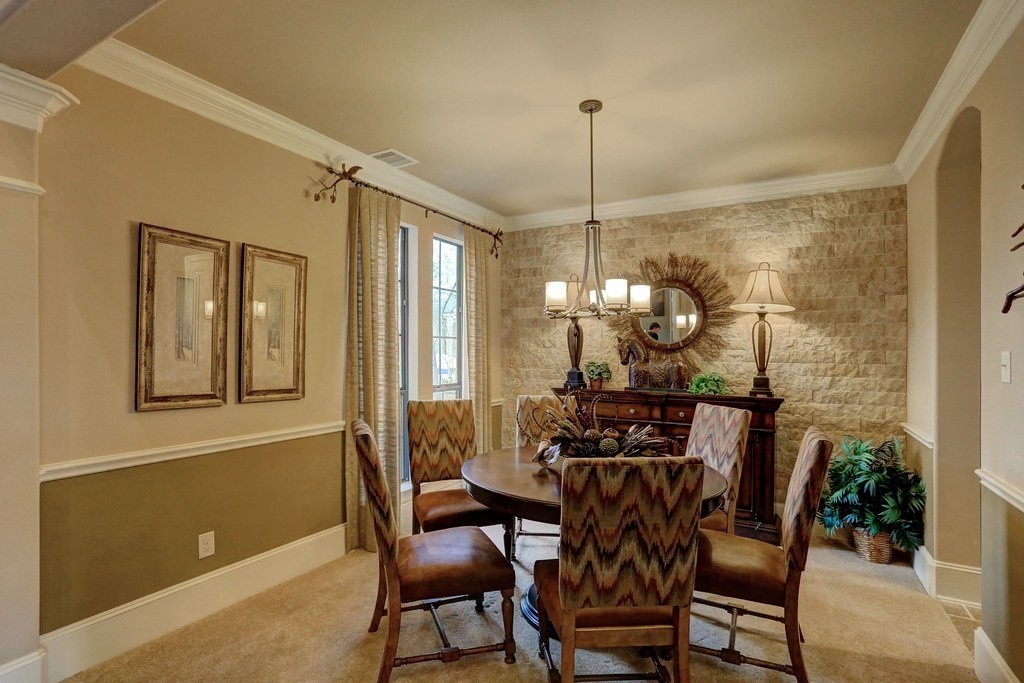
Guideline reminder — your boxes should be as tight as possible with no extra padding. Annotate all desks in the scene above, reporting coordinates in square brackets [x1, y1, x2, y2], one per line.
[461, 445, 730, 563]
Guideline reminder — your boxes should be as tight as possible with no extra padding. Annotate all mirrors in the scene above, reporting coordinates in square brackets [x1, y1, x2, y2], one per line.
[629, 277, 705, 351]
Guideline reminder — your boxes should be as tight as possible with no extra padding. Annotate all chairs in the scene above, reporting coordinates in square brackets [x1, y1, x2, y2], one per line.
[686, 402, 753, 535]
[694, 425, 834, 683]
[534, 456, 706, 683]
[350, 417, 520, 683]
[511, 395, 577, 561]
[409, 398, 512, 564]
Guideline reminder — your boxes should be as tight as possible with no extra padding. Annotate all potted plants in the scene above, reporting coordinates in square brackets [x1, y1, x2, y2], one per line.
[688, 372, 731, 396]
[584, 360, 612, 390]
[817, 434, 926, 565]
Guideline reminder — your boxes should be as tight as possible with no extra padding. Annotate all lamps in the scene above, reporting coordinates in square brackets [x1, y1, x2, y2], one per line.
[676, 314, 696, 329]
[543, 99, 655, 321]
[550, 273, 594, 389]
[732, 261, 797, 396]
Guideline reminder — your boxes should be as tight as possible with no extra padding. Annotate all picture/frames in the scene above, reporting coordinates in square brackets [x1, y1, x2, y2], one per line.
[133, 221, 232, 415]
[238, 241, 310, 403]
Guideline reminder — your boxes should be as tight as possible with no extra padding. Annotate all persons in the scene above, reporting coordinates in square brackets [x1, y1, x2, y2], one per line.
[648, 322, 661, 340]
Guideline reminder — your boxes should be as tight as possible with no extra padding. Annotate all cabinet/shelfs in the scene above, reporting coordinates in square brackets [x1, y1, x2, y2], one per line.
[550, 386, 786, 544]
